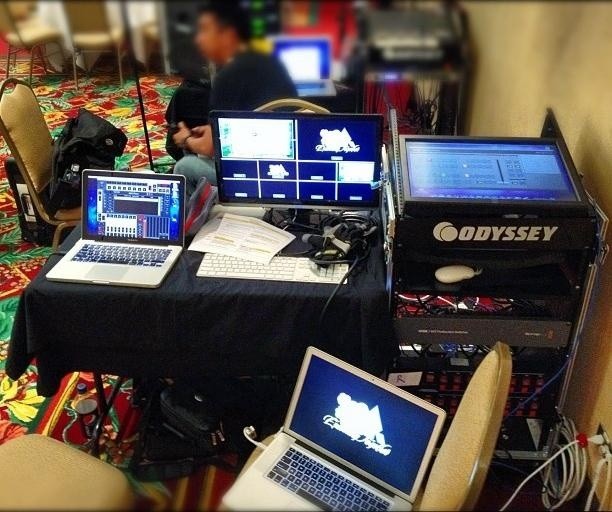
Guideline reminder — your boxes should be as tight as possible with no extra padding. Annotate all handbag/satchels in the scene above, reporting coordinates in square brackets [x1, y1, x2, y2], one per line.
[159, 380, 225, 449]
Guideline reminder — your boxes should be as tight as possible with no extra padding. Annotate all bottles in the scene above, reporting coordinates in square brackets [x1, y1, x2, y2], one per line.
[71, 384, 99, 441]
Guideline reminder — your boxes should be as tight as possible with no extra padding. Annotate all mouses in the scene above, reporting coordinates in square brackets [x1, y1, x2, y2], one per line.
[434, 264, 475, 284]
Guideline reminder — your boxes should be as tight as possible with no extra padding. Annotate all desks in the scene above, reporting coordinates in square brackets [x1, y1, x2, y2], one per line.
[5, 210, 382, 459]
[37, 0, 158, 75]
[165, 81, 356, 164]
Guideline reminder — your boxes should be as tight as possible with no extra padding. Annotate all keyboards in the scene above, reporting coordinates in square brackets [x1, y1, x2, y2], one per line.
[195, 249, 348, 284]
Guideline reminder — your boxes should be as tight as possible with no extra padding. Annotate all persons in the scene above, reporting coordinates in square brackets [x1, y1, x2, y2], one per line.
[175, 8, 297, 210]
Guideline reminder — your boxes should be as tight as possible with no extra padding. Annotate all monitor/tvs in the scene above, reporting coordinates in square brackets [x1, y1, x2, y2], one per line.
[206, 107, 385, 226]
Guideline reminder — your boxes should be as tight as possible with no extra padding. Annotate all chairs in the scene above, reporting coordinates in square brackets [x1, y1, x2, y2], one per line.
[219, 341, 512, 512]
[62, 0, 129, 89]
[0, 0, 67, 84]
[0, 433, 134, 512]
[0, 77, 82, 252]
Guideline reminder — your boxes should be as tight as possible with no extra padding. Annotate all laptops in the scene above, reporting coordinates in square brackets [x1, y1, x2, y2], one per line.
[46, 168, 185, 288]
[269, 36, 334, 95]
[220, 346, 446, 512]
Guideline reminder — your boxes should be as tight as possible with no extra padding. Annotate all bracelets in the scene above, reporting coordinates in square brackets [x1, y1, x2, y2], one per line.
[182, 135, 192, 150]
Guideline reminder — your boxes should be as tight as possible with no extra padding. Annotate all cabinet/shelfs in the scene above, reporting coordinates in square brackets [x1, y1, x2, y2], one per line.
[360, 57, 467, 138]
[380, 143, 610, 473]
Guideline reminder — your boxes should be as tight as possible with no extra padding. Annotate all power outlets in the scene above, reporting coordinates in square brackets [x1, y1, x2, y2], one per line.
[593, 422, 612, 466]
